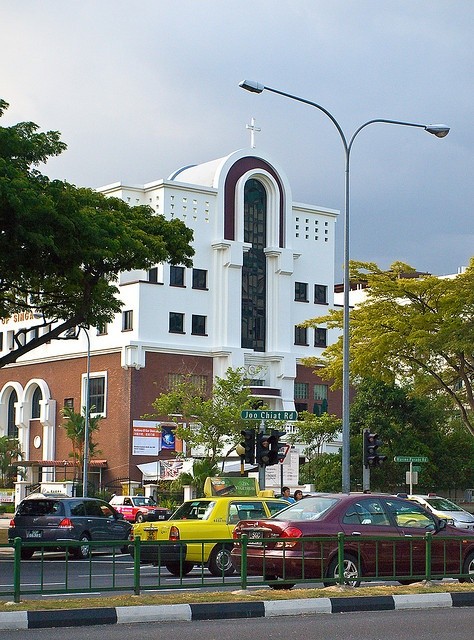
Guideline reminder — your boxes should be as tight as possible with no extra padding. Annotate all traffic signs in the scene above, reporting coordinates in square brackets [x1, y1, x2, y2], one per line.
[393, 455, 429, 463]
[239, 409, 299, 421]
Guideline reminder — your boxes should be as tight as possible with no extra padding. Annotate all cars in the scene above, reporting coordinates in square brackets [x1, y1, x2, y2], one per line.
[371, 492, 474, 531]
[229, 491, 474, 590]
[128, 497, 290, 575]
[99, 495, 171, 522]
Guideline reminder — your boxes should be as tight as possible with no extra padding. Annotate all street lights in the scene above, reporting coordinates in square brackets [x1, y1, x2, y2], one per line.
[233, 80, 449, 490]
[33, 312, 91, 498]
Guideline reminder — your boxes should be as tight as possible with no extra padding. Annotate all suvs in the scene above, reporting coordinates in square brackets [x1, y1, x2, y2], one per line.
[6, 497, 132, 559]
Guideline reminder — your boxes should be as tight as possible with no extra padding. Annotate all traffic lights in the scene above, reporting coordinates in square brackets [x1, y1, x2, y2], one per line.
[364, 429, 378, 469]
[256, 432, 274, 466]
[271, 430, 286, 464]
[239, 428, 254, 464]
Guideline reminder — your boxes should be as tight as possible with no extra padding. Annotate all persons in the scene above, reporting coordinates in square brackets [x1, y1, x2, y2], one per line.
[279, 487, 295, 505]
[294, 490, 303, 502]
